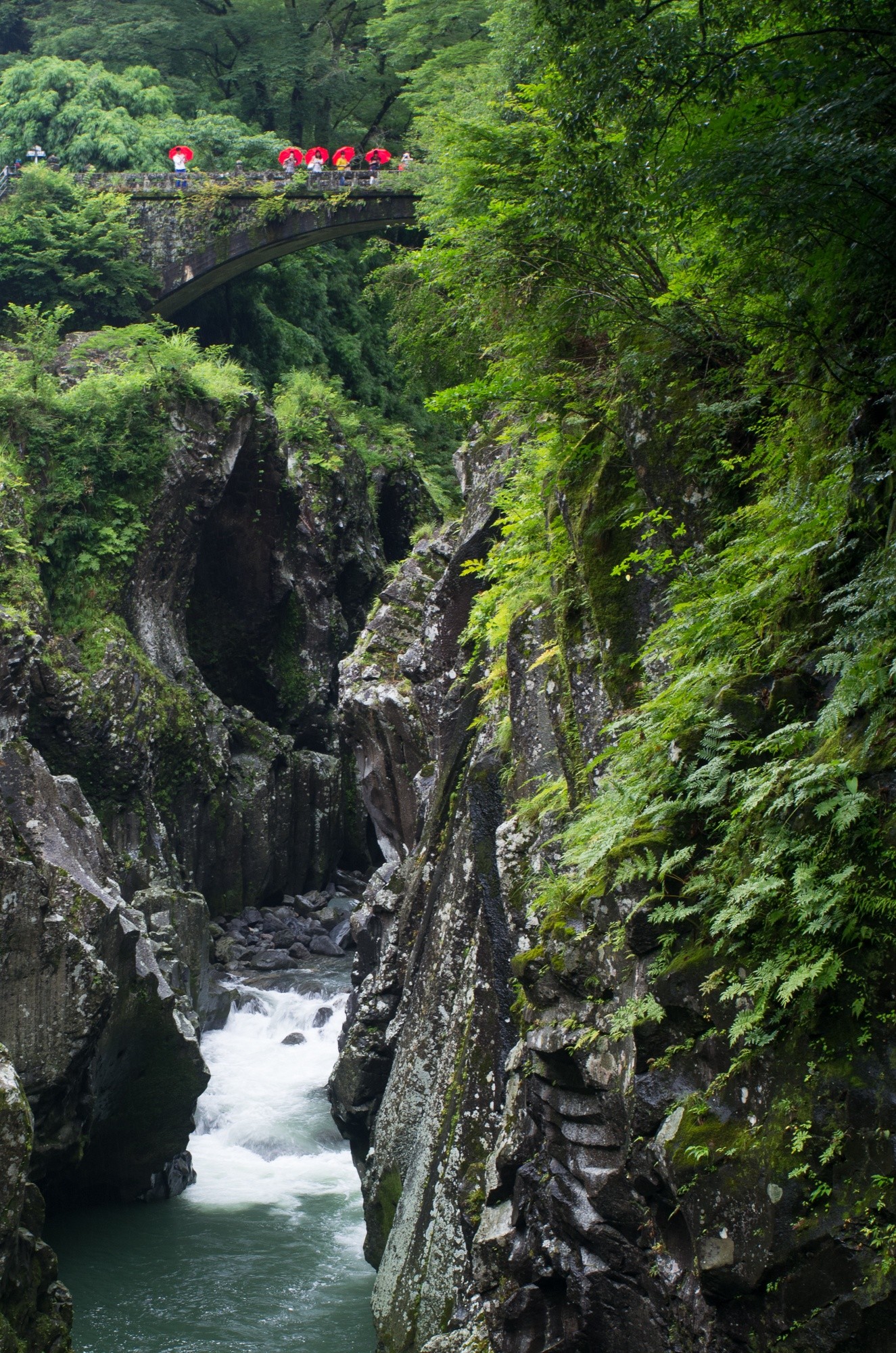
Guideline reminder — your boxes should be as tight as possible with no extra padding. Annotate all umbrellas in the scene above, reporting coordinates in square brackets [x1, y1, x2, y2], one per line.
[170, 146, 194, 163]
[304, 147, 329, 164]
[332, 146, 355, 165]
[278, 147, 303, 166]
[365, 148, 391, 164]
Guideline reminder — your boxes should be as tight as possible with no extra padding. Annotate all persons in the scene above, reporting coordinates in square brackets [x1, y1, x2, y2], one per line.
[14, 158, 22, 168]
[370, 150, 381, 171]
[336, 150, 351, 170]
[283, 151, 298, 182]
[307, 150, 323, 173]
[401, 152, 414, 169]
[173, 148, 188, 187]
